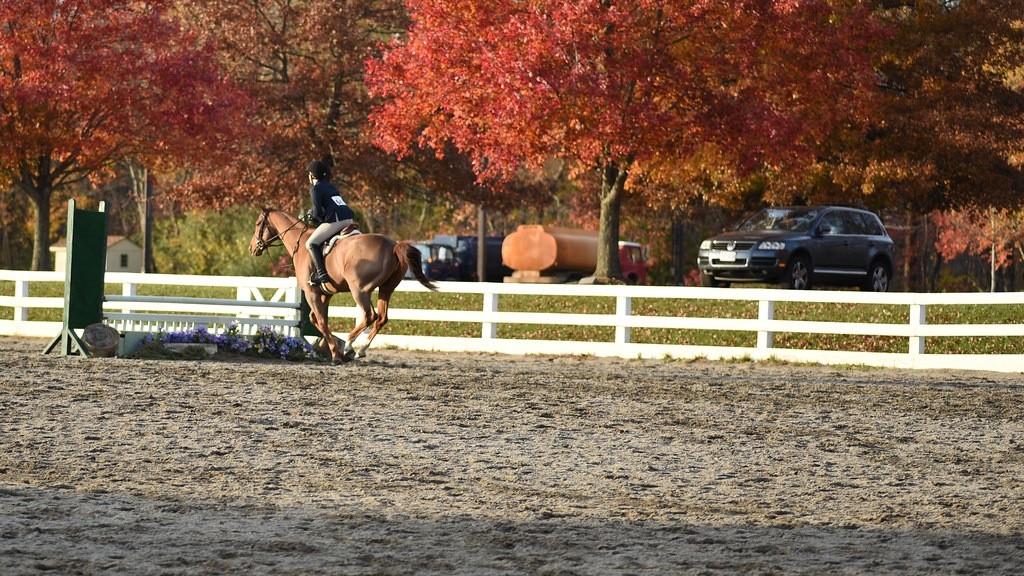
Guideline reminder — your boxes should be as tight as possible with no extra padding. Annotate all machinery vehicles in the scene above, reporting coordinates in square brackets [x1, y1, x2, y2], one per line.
[501, 225, 648, 285]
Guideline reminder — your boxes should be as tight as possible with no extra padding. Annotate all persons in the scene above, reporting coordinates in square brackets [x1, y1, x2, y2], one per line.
[306, 161, 357, 285]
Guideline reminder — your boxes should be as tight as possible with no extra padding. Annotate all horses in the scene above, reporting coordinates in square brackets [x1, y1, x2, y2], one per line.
[249, 201, 440, 367]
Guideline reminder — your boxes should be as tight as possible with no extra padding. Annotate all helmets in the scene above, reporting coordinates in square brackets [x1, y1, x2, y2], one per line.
[306, 160, 330, 180]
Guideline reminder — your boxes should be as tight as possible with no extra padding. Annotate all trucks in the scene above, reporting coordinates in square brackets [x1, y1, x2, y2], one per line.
[404, 235, 514, 282]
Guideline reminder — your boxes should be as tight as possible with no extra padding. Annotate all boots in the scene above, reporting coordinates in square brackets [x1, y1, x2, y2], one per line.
[308, 243, 330, 283]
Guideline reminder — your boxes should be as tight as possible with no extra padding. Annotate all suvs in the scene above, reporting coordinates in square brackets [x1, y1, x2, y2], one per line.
[697, 205, 897, 292]
